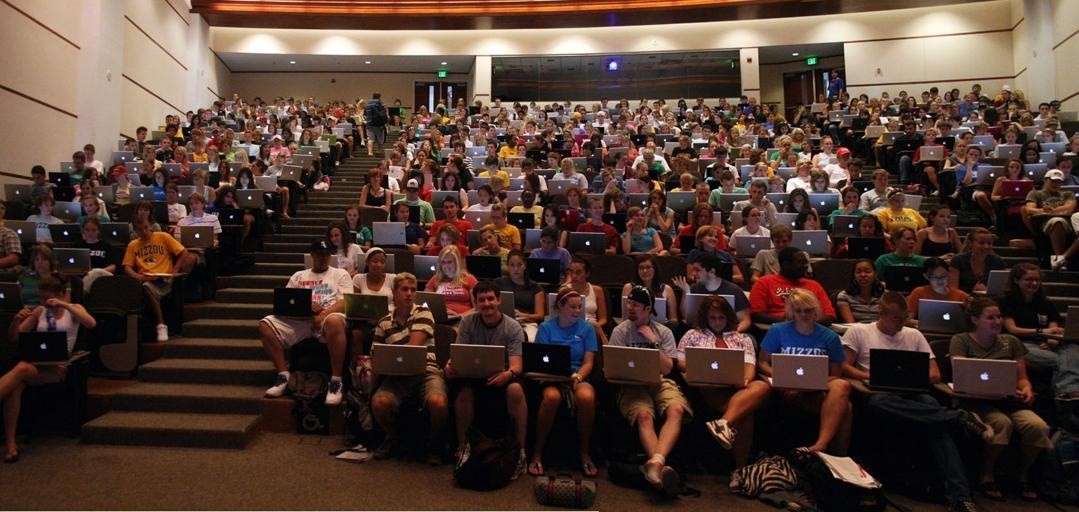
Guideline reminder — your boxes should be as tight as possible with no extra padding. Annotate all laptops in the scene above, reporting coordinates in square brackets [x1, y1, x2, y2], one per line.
[776, 213, 798, 230]
[52, 186, 75, 200]
[584, 113, 596, 122]
[501, 168, 521, 178]
[469, 190, 495, 206]
[1033, 120, 1046, 130]
[389, 205, 421, 222]
[667, 192, 696, 212]
[343, 294, 389, 321]
[360, 208, 386, 229]
[236, 189, 264, 208]
[730, 149, 739, 162]
[548, 293, 588, 322]
[848, 236, 885, 258]
[693, 143, 708, 155]
[332, 128, 343, 139]
[470, 291, 528, 323]
[593, 148, 602, 160]
[99, 222, 131, 244]
[852, 118, 870, 130]
[189, 163, 209, 174]
[630, 194, 651, 208]
[180, 226, 213, 250]
[973, 271, 1012, 296]
[466, 212, 492, 229]
[52, 248, 92, 275]
[1040, 142, 1067, 160]
[551, 117, 561, 124]
[507, 157, 525, 165]
[569, 233, 606, 253]
[94, 186, 113, 203]
[809, 194, 838, 216]
[830, 215, 861, 237]
[573, 157, 586, 172]
[48, 223, 87, 244]
[496, 128, 506, 136]
[254, 176, 277, 192]
[1029, 212, 1072, 223]
[995, 144, 1022, 160]
[971, 135, 993, 152]
[467, 231, 500, 254]
[747, 135, 759, 145]
[648, 169, 658, 180]
[548, 180, 571, 197]
[720, 194, 750, 213]
[149, 202, 169, 224]
[916, 130, 926, 137]
[470, 128, 480, 136]
[415, 149, 422, 156]
[314, 140, 329, 153]
[768, 194, 791, 212]
[553, 149, 571, 158]
[130, 188, 153, 204]
[3, 184, 32, 203]
[769, 354, 828, 390]
[113, 151, 133, 164]
[767, 148, 780, 160]
[510, 120, 523, 127]
[913, 299, 967, 335]
[373, 345, 428, 376]
[473, 177, 490, 190]
[918, 147, 944, 166]
[526, 150, 547, 161]
[778, 168, 796, 180]
[962, 122, 978, 132]
[1038, 305, 1079, 341]
[603, 135, 621, 146]
[301, 146, 320, 158]
[440, 148, 453, 158]
[473, 157, 489, 172]
[602, 213, 626, 234]
[563, 109, 570, 115]
[735, 158, 749, 171]
[613, 296, 666, 326]
[654, 134, 673, 145]
[465, 146, 485, 156]
[508, 192, 524, 208]
[509, 179, 524, 190]
[277, 165, 302, 181]
[968, 166, 1005, 189]
[665, 142, 681, 155]
[862, 348, 930, 394]
[118, 140, 139, 153]
[882, 132, 903, 145]
[706, 167, 729, 176]
[575, 134, 588, 146]
[0, 282, 23, 316]
[260, 134, 271, 143]
[519, 135, 533, 142]
[1038, 153, 1056, 168]
[852, 181, 875, 194]
[3, 220, 38, 244]
[521, 341, 571, 377]
[609, 109, 619, 115]
[321, 134, 336, 145]
[612, 115, 620, 122]
[735, 236, 770, 258]
[684, 346, 744, 387]
[450, 344, 506, 379]
[829, 110, 846, 121]
[595, 127, 603, 135]
[902, 196, 922, 210]
[792, 230, 828, 258]
[508, 213, 535, 229]
[963, 144, 985, 161]
[414, 292, 461, 325]
[608, 147, 627, 162]
[526, 228, 561, 250]
[741, 166, 755, 183]
[389, 166, 403, 176]
[231, 147, 249, 156]
[866, 126, 885, 138]
[372, 222, 406, 246]
[997, 180, 1034, 199]
[229, 163, 241, 177]
[152, 131, 165, 141]
[751, 177, 770, 192]
[417, 141, 425, 149]
[843, 115, 858, 127]
[465, 255, 501, 281]
[1023, 126, 1039, 141]
[16, 331, 70, 362]
[162, 163, 182, 177]
[812, 104, 826, 112]
[414, 255, 439, 279]
[124, 162, 143, 175]
[339, 123, 352, 135]
[885, 265, 922, 291]
[52, 201, 81, 220]
[127, 174, 140, 186]
[1022, 164, 1047, 185]
[947, 357, 1018, 397]
[683, 294, 734, 326]
[526, 258, 560, 284]
[432, 190, 458, 209]
[274, 287, 317, 317]
[48, 172, 70, 186]
[421, 129, 430, 135]
[951, 129, 969, 139]
[688, 212, 721, 225]
[304, 254, 338, 270]
[1059, 185, 1079, 196]
[587, 193, 604, 205]
[602, 345, 660, 387]
[692, 133, 703, 140]
[680, 235, 696, 254]
[529, 113, 539, 119]
[389, 107, 400, 114]
[759, 138, 774, 150]
[631, 134, 646, 145]
[469, 106, 480, 114]
[732, 211, 764, 233]
[440, 166, 447, 176]
[293, 154, 313, 169]
[701, 159, 718, 173]
[489, 108, 500, 115]
[182, 127, 193, 136]
[177, 186, 196, 204]
[357, 253, 394, 274]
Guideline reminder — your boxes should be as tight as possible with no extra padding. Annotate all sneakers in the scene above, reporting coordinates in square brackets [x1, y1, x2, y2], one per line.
[661, 466, 677, 495]
[929, 188, 940, 197]
[324, 375, 343, 406]
[455, 442, 471, 470]
[157, 323, 168, 341]
[639, 460, 662, 485]
[374, 437, 392, 459]
[1059, 263, 1069, 270]
[264, 371, 290, 397]
[360, 140, 365, 146]
[510, 457, 528, 480]
[427, 447, 441, 466]
[706, 418, 739, 450]
[1050, 254, 1065, 269]
[335, 160, 340, 166]
[958, 501, 976, 512]
[963, 411, 994, 444]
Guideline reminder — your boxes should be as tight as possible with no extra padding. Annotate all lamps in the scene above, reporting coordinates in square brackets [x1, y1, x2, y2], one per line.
[807, 57, 816, 66]
[438, 70, 447, 79]
[608, 59, 617, 71]
[731, 60, 734, 69]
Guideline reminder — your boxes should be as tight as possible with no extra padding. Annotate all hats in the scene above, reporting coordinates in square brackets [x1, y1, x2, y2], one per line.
[1002, 83, 1011, 92]
[111, 165, 126, 176]
[406, 178, 419, 188]
[836, 146, 851, 158]
[309, 238, 337, 251]
[1044, 169, 1065, 181]
[628, 285, 658, 317]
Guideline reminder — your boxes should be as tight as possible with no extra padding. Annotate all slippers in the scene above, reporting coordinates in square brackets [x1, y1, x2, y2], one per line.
[982, 485, 1006, 502]
[1016, 484, 1041, 502]
[581, 460, 598, 477]
[527, 460, 544, 477]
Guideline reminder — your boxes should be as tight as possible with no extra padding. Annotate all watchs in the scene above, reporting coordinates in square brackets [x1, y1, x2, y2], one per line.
[575, 374, 583, 382]
[650, 340, 661, 348]
[508, 369, 517, 379]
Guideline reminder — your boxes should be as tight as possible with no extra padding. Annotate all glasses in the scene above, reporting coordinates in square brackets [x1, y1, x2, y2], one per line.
[794, 308, 815, 314]
[750, 212, 761, 218]
[638, 265, 653, 270]
[928, 273, 950, 280]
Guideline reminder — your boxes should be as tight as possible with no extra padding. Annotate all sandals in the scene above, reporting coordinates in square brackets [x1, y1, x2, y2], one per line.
[3, 444, 19, 463]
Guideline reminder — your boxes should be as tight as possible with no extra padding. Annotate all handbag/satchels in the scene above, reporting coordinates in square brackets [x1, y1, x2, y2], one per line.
[533, 475, 595, 508]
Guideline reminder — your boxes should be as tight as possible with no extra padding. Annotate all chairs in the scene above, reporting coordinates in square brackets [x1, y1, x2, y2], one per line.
[928, 338, 953, 385]
[430, 323, 457, 371]
[87, 275, 145, 377]
[588, 253, 635, 321]
[674, 252, 688, 275]
[813, 259, 859, 298]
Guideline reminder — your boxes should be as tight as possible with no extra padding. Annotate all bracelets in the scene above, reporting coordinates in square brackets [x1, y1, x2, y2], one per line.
[1036, 327, 1044, 333]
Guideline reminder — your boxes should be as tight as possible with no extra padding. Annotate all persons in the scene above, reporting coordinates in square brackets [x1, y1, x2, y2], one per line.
[1023, 139, 1041, 152]
[632, 161, 661, 190]
[266, 151, 306, 189]
[235, 149, 249, 166]
[837, 259, 889, 324]
[226, 91, 257, 132]
[121, 213, 187, 341]
[748, 148, 775, 178]
[974, 120, 991, 135]
[534, 104, 579, 153]
[462, 183, 507, 223]
[494, 249, 545, 342]
[424, 246, 479, 335]
[359, 168, 392, 222]
[257, 95, 305, 157]
[708, 171, 749, 211]
[1062, 134, 1079, 156]
[488, 204, 520, 252]
[346, 247, 397, 375]
[449, 142, 473, 170]
[912, 130, 952, 196]
[165, 115, 180, 136]
[84, 144, 104, 176]
[582, 142, 601, 176]
[670, 173, 697, 192]
[496, 102, 541, 157]
[420, 160, 439, 190]
[18, 244, 56, 310]
[799, 140, 815, 163]
[392, 201, 426, 254]
[603, 189, 625, 214]
[426, 223, 470, 257]
[1024, 169, 1079, 271]
[906, 258, 972, 327]
[112, 166, 135, 206]
[68, 151, 88, 185]
[678, 182, 728, 232]
[670, 202, 727, 254]
[174, 192, 222, 278]
[768, 103, 818, 136]
[622, 254, 676, 322]
[732, 144, 752, 165]
[950, 297, 1050, 500]
[26, 193, 64, 241]
[257, 237, 353, 405]
[729, 95, 770, 144]
[428, 196, 473, 244]
[859, 169, 897, 211]
[783, 188, 821, 229]
[621, 207, 669, 256]
[473, 224, 512, 277]
[825, 147, 853, 187]
[529, 287, 598, 477]
[206, 184, 239, 214]
[251, 160, 290, 218]
[827, 187, 873, 249]
[948, 115, 974, 134]
[812, 135, 838, 170]
[998, 122, 1026, 145]
[641, 190, 676, 242]
[787, 153, 798, 167]
[166, 184, 188, 233]
[509, 159, 521, 168]
[816, 94, 825, 125]
[411, 150, 427, 170]
[991, 157, 1036, 236]
[72, 179, 108, 216]
[671, 254, 752, 333]
[843, 90, 873, 153]
[705, 164, 725, 184]
[299, 95, 367, 175]
[559, 186, 592, 223]
[387, 178, 436, 236]
[895, 84, 1019, 120]
[362, 91, 387, 156]
[558, 259, 608, 345]
[1022, 148, 1040, 164]
[943, 140, 967, 199]
[236, 132, 260, 156]
[340, 204, 372, 250]
[836, 215, 892, 257]
[236, 166, 260, 252]
[192, 139, 208, 162]
[392, 141, 410, 170]
[170, 146, 192, 175]
[121, 127, 154, 153]
[612, 150, 634, 178]
[743, 161, 769, 188]
[786, 158, 815, 193]
[220, 140, 235, 162]
[83, 165, 99, 186]
[516, 158, 548, 194]
[1035, 103, 1053, 119]
[576, 198, 620, 255]
[0, 199, 6, 227]
[420, 140, 437, 159]
[574, 97, 637, 148]
[1041, 128, 1057, 143]
[875, 225, 930, 291]
[677, 296, 770, 469]
[478, 156, 511, 188]
[608, 285, 693, 489]
[1018, 112, 1034, 126]
[699, 136, 729, 163]
[994, 127, 1021, 157]
[961, 132, 974, 143]
[796, 208, 832, 257]
[443, 154, 475, 189]
[911, 204, 961, 257]
[147, 167, 169, 200]
[730, 181, 778, 232]
[805, 169, 844, 210]
[439, 172, 469, 212]
[829, 70, 842, 110]
[635, 97, 681, 143]
[705, 147, 740, 184]
[937, 123, 960, 141]
[837, 157, 868, 193]
[31, 166, 56, 193]
[368, 272, 446, 465]
[677, 97, 731, 137]
[770, 135, 799, 169]
[757, 288, 852, 468]
[728, 206, 774, 256]
[402, 170, 432, 204]
[70, 218, 117, 303]
[76, 195, 111, 226]
[390, 151, 401, 166]
[534, 203, 568, 248]
[844, 291, 993, 512]
[897, 121, 924, 186]
[686, 226, 745, 284]
[870, 91, 898, 170]
[548, 153, 561, 169]
[327, 221, 364, 279]
[829, 102, 842, 146]
[1002, 261, 1079, 431]
[377, 160, 399, 192]
[530, 226, 571, 285]
[672, 135, 698, 162]
[1056, 157, 1079, 197]
[750, 247, 837, 325]
[0, 227, 24, 274]
[188, 169, 216, 205]
[206, 146, 218, 171]
[791, 128, 809, 151]
[1035, 116, 1069, 144]
[666, 154, 700, 183]
[429, 99, 450, 141]
[154, 137, 174, 161]
[225, 128, 240, 147]
[445, 281, 530, 481]
[956, 146, 995, 223]
[218, 160, 237, 186]
[474, 99, 500, 155]
[948, 227, 1009, 297]
[1051, 100, 1061, 117]
[137, 159, 155, 185]
[180, 101, 225, 145]
[387, 99, 430, 141]
[921, 115, 934, 130]
[875, 187, 927, 239]
[523, 173, 548, 206]
[552, 158, 588, 194]
[450, 97, 473, 146]
[1, 274, 96, 463]
[598, 169, 626, 192]
[137, 145, 162, 171]
[509, 188, 545, 229]
[632, 141, 671, 174]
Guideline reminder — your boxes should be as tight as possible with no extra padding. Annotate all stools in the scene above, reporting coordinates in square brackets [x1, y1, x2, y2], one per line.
[32, 349, 92, 441]
[936, 158, 958, 202]
